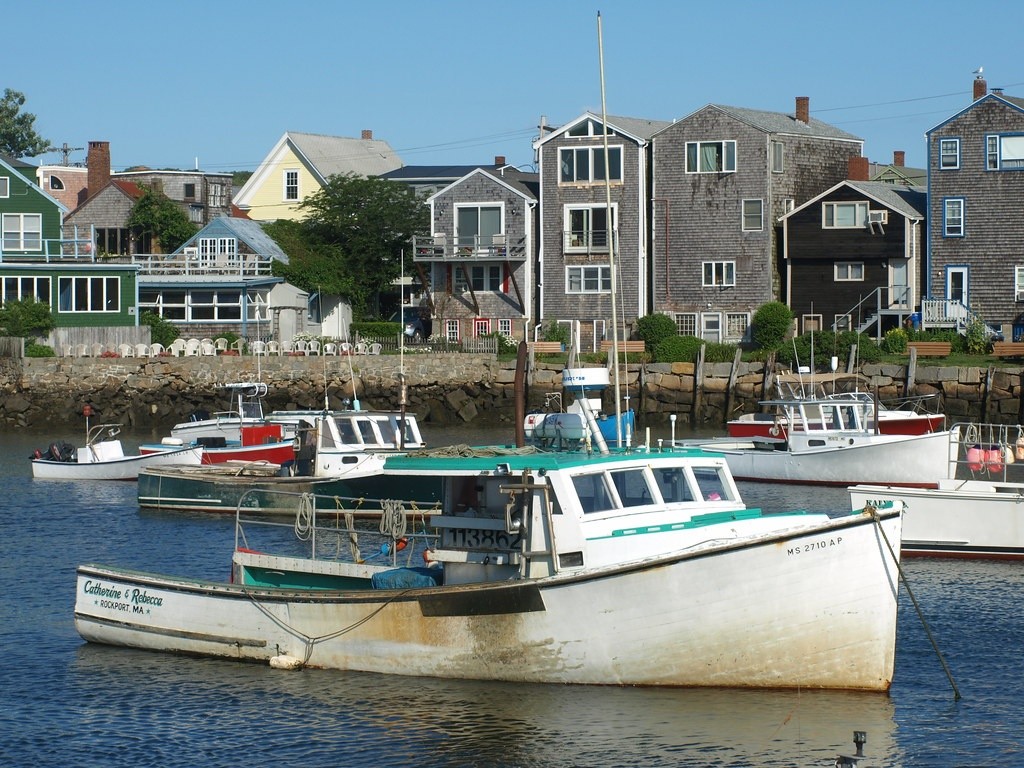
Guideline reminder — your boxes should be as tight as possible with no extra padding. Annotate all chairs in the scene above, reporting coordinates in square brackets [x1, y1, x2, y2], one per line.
[60, 337, 386, 358]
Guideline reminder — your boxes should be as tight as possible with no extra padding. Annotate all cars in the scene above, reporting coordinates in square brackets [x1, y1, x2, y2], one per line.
[386, 306, 433, 345]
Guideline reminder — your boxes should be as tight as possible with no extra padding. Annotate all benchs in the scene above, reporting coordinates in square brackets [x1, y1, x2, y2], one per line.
[989, 341, 1024, 360]
[902, 341, 952, 355]
[525, 341, 562, 356]
[601, 341, 645, 353]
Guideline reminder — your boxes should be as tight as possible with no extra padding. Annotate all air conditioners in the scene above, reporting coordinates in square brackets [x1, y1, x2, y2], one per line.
[868, 209, 888, 225]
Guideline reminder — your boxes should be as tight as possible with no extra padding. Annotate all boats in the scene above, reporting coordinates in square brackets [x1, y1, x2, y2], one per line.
[725, 351, 947, 436]
[520, 332, 636, 442]
[28, 404, 205, 481]
[73, 319, 905, 694]
[137, 378, 444, 522]
[138, 380, 301, 468]
[653, 301, 960, 493]
[842, 478, 1024, 565]
